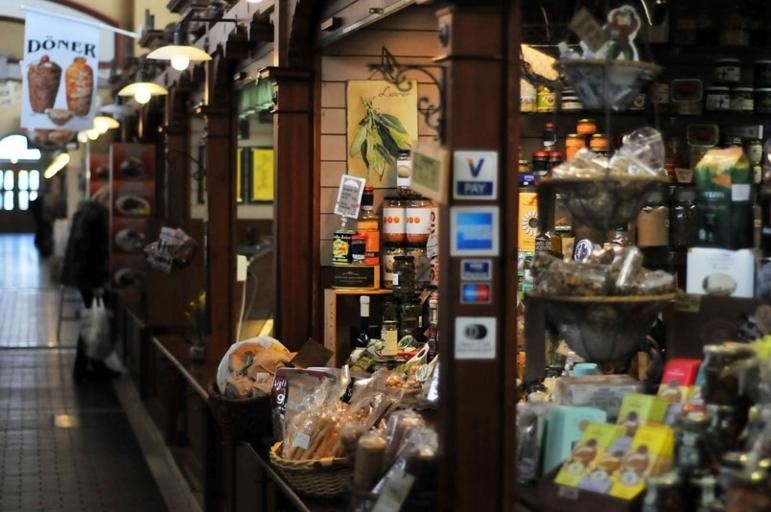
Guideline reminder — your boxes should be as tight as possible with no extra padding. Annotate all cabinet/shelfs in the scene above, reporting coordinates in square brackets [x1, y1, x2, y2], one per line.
[136, 328, 209, 511]
[111, 305, 138, 403]
[219, 412, 438, 512]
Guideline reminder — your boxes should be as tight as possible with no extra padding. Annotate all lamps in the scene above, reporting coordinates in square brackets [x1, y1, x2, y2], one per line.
[146, 7, 237, 71]
[118, 62, 169, 104]
[94, 114, 120, 130]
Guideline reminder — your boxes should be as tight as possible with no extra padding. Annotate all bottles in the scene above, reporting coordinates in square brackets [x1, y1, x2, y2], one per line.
[752, 162, 762, 184]
[565, 133, 586, 148]
[392, 255, 416, 299]
[754, 60, 771, 88]
[636, 190, 671, 248]
[714, 58, 743, 86]
[697, 345, 759, 404]
[543, 122, 558, 147]
[755, 88, 771, 111]
[730, 87, 755, 111]
[400, 298, 424, 330]
[548, 150, 564, 171]
[347, 295, 375, 374]
[380, 320, 399, 356]
[396, 149, 421, 197]
[671, 189, 701, 247]
[589, 133, 611, 147]
[576, 118, 597, 134]
[406, 197, 433, 243]
[537, 84, 560, 112]
[405, 243, 431, 291]
[520, 77, 537, 113]
[356, 206, 380, 231]
[519, 160, 530, 186]
[382, 298, 400, 328]
[604, 245, 644, 295]
[704, 403, 748, 467]
[532, 150, 548, 171]
[382, 197, 406, 242]
[361, 185, 375, 206]
[382, 242, 405, 290]
[642, 473, 683, 512]
[672, 410, 716, 476]
[705, 85, 730, 112]
[746, 140, 763, 162]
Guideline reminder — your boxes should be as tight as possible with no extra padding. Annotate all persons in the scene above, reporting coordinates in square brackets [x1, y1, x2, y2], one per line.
[60, 186, 116, 384]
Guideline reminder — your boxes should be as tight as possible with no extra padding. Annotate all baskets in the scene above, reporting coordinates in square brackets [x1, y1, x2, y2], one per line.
[269, 438, 348, 495]
[206, 381, 270, 424]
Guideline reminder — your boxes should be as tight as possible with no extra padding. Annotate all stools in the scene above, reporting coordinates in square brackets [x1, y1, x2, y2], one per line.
[56, 282, 99, 342]
